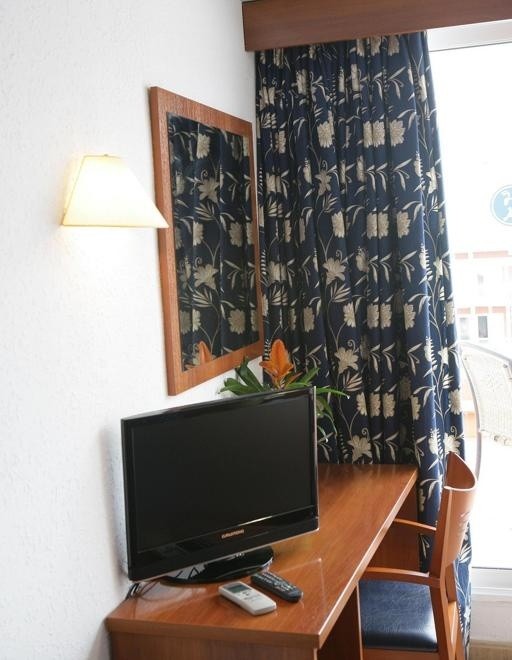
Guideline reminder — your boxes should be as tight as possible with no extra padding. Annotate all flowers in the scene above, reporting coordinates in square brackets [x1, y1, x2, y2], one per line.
[218, 338, 347, 463]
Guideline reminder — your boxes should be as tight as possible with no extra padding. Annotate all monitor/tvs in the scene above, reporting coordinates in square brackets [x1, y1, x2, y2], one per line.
[120, 384, 321, 585]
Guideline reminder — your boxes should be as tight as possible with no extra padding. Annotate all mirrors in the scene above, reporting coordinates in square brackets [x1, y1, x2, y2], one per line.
[147, 85, 265, 398]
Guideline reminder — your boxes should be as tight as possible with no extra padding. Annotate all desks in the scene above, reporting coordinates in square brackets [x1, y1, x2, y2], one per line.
[104, 461, 426, 660]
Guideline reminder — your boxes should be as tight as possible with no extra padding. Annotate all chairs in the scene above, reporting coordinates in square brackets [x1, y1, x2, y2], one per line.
[331, 450, 478, 659]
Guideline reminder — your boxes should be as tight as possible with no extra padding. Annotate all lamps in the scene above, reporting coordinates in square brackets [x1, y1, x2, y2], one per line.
[60, 153, 171, 230]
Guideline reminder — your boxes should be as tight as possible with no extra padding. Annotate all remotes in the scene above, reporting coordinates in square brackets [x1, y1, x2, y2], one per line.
[217, 580, 277, 616]
[250, 570, 304, 603]
